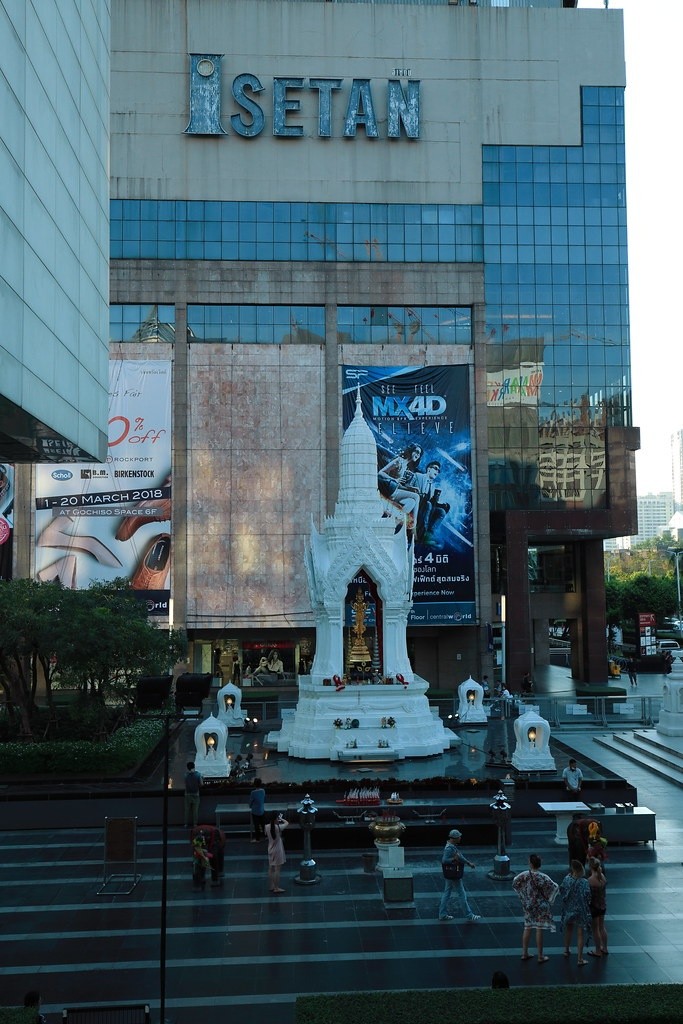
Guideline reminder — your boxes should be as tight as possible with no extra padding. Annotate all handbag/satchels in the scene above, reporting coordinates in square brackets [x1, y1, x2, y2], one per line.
[442, 846, 464, 879]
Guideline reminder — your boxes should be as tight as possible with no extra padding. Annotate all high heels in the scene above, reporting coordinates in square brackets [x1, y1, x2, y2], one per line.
[37, 555, 76, 590]
[35, 512, 124, 569]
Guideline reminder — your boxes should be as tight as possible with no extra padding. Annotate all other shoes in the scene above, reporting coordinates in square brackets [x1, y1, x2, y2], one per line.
[268, 887, 286, 892]
[184, 823, 189, 827]
[252, 839, 261, 843]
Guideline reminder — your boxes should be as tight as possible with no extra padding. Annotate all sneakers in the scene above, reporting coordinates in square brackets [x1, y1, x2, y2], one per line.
[438, 915, 454, 920]
[467, 915, 481, 921]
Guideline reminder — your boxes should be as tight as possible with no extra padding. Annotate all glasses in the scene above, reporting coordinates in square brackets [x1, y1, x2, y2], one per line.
[429, 466, 441, 474]
[413, 450, 422, 457]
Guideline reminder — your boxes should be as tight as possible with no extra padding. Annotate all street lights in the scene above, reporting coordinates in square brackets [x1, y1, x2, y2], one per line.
[500, 593, 506, 687]
[130, 669, 212, 1024]
[667, 545, 683, 643]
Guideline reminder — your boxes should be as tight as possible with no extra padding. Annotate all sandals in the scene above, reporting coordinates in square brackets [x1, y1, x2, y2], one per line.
[577, 959, 589, 966]
[564, 950, 571, 957]
[521, 953, 534, 959]
[538, 956, 549, 963]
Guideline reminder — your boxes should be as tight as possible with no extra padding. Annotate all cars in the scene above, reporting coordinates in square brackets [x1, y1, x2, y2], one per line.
[548, 620, 569, 639]
[656, 639, 680, 654]
[665, 618, 683, 631]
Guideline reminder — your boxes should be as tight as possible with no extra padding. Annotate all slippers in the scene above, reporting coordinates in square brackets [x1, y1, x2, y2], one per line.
[587, 950, 602, 957]
[601, 949, 608, 954]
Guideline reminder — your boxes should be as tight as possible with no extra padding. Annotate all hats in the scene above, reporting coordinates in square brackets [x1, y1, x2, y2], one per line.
[449, 829, 462, 838]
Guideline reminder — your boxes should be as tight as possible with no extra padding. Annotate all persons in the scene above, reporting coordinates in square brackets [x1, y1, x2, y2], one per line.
[494, 680, 502, 692]
[567, 813, 600, 868]
[560, 860, 591, 965]
[232, 661, 242, 686]
[249, 778, 265, 843]
[265, 811, 289, 892]
[628, 658, 637, 686]
[588, 857, 609, 956]
[232, 652, 238, 661]
[299, 658, 312, 675]
[253, 650, 284, 674]
[245, 666, 263, 687]
[352, 593, 367, 639]
[512, 854, 559, 962]
[405, 461, 447, 544]
[185, 762, 203, 828]
[378, 443, 423, 540]
[524, 672, 533, 694]
[501, 686, 510, 698]
[439, 830, 481, 922]
[563, 760, 584, 802]
[481, 676, 489, 698]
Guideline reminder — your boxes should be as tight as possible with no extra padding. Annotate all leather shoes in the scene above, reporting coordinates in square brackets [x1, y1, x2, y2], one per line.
[118, 479, 172, 541]
[129, 534, 171, 590]
